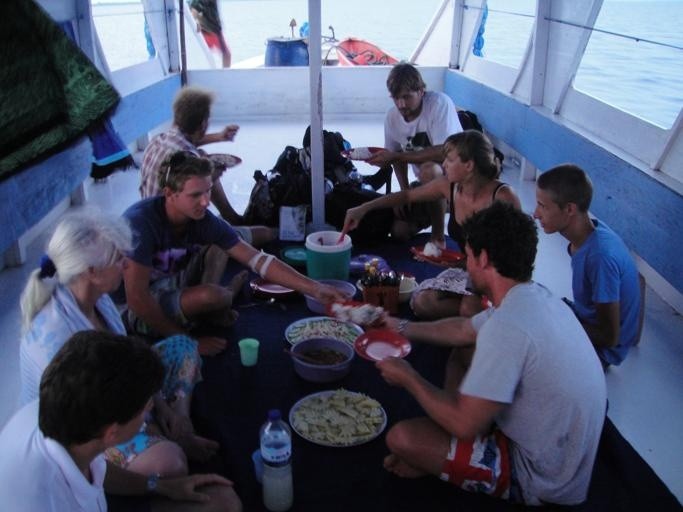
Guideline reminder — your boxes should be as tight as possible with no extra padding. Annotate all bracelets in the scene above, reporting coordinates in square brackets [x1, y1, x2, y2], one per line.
[145, 471, 165, 497]
[396, 318, 409, 337]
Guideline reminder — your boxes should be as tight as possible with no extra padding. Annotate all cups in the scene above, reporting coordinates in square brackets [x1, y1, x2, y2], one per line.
[251, 448, 262, 482]
[238, 337, 260, 367]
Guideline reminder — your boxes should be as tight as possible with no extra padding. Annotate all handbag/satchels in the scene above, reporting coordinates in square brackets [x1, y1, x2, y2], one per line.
[250, 146, 312, 227]
[302, 124, 358, 184]
[325, 182, 396, 257]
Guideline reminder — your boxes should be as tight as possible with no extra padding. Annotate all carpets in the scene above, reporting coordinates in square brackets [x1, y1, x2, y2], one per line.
[107, 232, 681, 511]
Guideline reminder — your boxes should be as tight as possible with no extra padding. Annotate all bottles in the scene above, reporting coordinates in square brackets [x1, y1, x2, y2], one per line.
[259, 409, 293, 511]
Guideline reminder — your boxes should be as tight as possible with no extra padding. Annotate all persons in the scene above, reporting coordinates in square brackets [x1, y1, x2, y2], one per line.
[364, 63, 466, 254]
[1, 328, 244, 511]
[138, 85, 270, 251]
[343, 130, 523, 319]
[189, 0, 232, 69]
[18, 204, 233, 482]
[374, 200, 607, 507]
[107, 150, 354, 358]
[532, 163, 642, 375]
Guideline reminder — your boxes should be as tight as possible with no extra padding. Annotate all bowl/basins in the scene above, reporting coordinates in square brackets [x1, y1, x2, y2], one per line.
[304, 279, 356, 313]
[290, 337, 353, 382]
[356, 276, 418, 302]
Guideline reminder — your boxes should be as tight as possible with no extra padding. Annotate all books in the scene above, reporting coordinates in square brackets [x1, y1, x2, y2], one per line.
[278, 204, 307, 242]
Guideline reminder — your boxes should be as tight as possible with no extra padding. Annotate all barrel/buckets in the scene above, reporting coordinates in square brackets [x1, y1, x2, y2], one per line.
[264, 36, 310, 67]
[303, 230, 354, 281]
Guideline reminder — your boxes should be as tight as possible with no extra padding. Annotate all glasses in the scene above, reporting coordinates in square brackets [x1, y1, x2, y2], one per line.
[165, 150, 200, 185]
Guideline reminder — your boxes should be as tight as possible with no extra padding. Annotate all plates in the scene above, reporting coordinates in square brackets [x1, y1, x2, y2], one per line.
[249, 277, 296, 293]
[354, 330, 412, 362]
[325, 299, 388, 327]
[285, 316, 364, 345]
[205, 153, 241, 166]
[289, 389, 387, 448]
[411, 244, 465, 263]
[339, 146, 388, 160]
